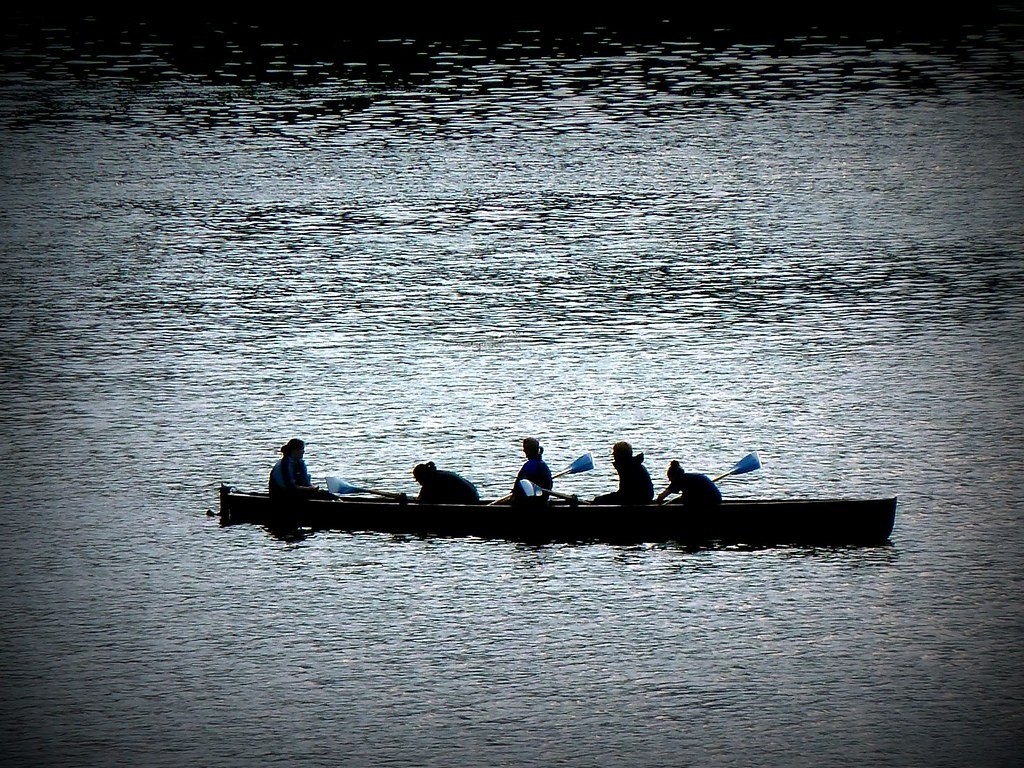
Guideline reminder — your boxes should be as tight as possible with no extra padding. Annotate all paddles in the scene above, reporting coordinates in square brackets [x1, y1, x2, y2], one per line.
[520, 479, 592, 505]
[488, 453, 594, 506]
[663, 451, 761, 508]
[324, 476, 419, 502]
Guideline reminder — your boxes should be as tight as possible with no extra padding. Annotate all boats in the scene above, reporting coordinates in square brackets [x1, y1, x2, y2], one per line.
[218, 480, 897, 547]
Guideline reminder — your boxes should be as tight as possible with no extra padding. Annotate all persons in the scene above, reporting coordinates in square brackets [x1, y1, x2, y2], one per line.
[268, 438, 342, 501]
[412, 462, 480, 506]
[655, 460, 721, 505]
[592, 442, 654, 504]
[513, 437, 554, 504]
